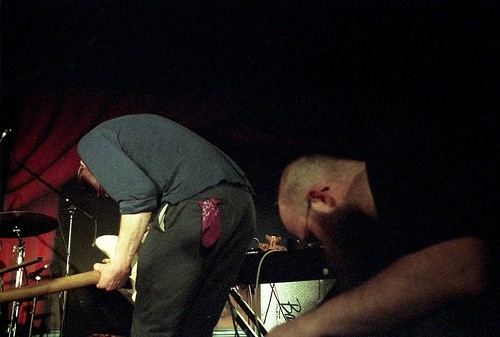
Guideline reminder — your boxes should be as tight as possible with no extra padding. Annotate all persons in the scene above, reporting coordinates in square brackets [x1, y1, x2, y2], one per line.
[265, 154, 500, 337]
[76, 114, 256, 337]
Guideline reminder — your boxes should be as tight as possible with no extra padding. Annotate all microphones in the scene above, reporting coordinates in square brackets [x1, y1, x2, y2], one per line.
[28, 264, 49, 279]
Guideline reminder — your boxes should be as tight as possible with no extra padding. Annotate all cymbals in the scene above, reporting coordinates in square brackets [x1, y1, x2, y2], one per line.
[0, 211, 59, 238]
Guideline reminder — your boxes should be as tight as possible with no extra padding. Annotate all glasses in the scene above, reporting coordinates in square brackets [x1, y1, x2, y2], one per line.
[288, 187, 330, 257]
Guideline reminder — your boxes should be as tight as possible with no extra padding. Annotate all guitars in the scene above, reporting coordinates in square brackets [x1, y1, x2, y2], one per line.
[0, 230, 149, 304]
